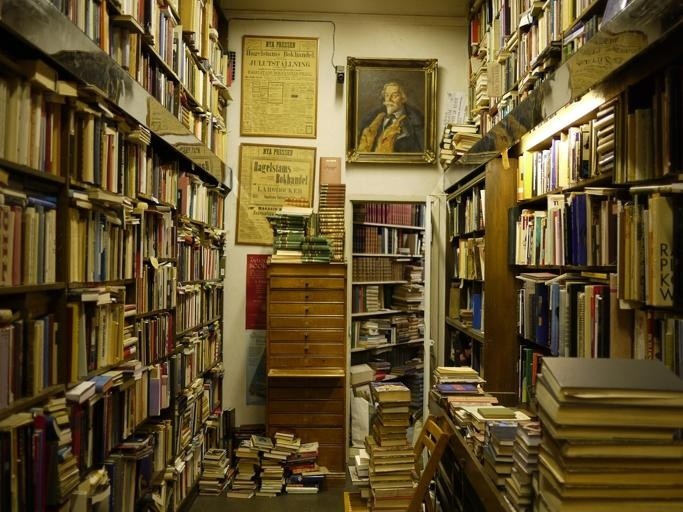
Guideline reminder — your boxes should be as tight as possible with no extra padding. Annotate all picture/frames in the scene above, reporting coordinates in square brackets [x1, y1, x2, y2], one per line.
[343, 55, 438, 166]
[352, 380, 375, 408]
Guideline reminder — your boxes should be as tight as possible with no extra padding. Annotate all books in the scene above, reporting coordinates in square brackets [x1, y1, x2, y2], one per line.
[427, 0, 683, 512]
[1, 1, 348, 511]
[348, 199, 427, 511]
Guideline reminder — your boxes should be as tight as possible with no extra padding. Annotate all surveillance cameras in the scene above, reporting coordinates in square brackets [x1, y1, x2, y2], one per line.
[335, 63, 345, 84]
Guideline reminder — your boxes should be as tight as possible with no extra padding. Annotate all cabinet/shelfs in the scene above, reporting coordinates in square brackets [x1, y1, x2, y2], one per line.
[352, 200, 425, 383]
[264, 256, 348, 481]
[1, 0, 225, 512]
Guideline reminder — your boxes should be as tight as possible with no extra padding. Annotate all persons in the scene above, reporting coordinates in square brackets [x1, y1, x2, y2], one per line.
[358, 80, 425, 153]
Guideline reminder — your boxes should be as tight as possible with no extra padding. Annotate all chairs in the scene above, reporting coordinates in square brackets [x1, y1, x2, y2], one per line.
[345, 421, 447, 511]
